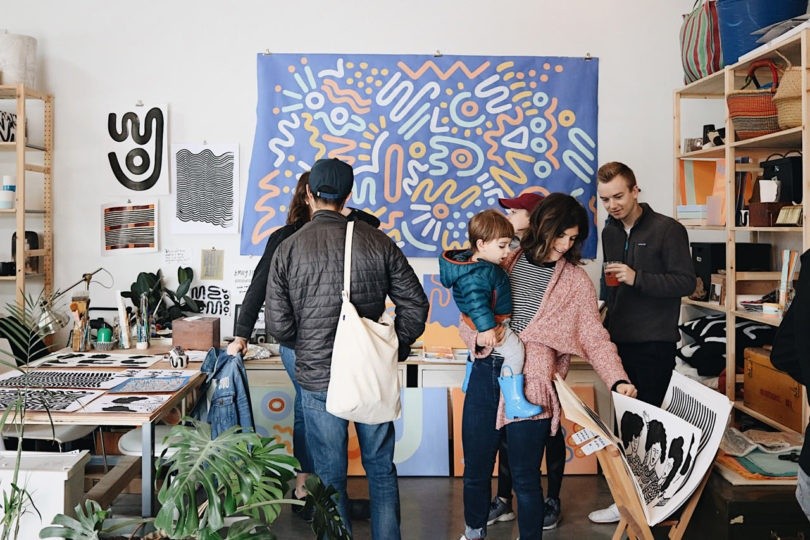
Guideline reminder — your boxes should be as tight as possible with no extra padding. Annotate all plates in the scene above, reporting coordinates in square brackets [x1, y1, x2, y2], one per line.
[740, 302, 761, 311]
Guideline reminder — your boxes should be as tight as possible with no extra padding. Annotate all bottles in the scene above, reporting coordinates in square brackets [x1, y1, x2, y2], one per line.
[0, 190, 14, 208]
[3, 175, 16, 204]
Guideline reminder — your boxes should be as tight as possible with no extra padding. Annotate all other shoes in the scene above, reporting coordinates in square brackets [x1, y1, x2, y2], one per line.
[290, 488, 315, 523]
[347, 498, 371, 522]
[588, 503, 621, 524]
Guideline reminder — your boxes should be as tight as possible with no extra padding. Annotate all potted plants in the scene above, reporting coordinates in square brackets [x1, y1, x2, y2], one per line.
[38, 417, 353, 540]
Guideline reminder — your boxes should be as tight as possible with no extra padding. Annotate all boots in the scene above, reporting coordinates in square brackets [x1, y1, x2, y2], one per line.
[497, 365, 543, 419]
[462, 351, 474, 393]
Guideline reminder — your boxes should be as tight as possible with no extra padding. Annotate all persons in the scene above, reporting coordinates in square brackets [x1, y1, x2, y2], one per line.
[589, 162, 697, 523]
[266, 158, 431, 540]
[456, 192, 638, 540]
[771, 247, 810, 540]
[440, 210, 544, 419]
[226, 171, 382, 518]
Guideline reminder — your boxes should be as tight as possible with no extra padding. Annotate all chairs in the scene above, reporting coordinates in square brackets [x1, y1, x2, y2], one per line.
[118, 351, 241, 483]
[0, 339, 107, 488]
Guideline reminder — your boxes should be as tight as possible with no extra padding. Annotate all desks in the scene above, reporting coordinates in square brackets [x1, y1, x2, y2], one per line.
[0, 334, 215, 519]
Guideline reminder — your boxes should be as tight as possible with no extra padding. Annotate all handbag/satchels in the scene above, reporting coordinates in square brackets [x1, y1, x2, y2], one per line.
[748, 150, 805, 228]
[679, 0, 721, 85]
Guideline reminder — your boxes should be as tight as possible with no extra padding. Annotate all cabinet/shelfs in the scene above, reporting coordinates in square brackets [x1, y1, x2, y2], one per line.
[244, 349, 614, 441]
[673, 22, 810, 442]
[0, 82, 55, 354]
[0, 450, 92, 540]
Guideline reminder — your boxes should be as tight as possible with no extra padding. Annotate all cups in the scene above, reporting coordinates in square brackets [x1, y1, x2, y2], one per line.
[169, 350, 188, 369]
[603, 261, 623, 286]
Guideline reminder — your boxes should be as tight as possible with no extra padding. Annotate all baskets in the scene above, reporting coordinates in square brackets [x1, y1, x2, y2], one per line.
[726, 59, 780, 141]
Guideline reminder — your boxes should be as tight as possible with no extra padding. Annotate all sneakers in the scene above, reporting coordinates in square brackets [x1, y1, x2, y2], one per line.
[486, 495, 516, 526]
[541, 496, 563, 530]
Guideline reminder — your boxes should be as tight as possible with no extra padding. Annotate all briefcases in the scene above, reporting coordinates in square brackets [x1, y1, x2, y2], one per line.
[325, 220, 402, 425]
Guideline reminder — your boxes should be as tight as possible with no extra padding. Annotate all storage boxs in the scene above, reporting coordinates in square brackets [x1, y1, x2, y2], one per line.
[744, 348, 803, 433]
[750, 201, 789, 227]
[172, 316, 220, 353]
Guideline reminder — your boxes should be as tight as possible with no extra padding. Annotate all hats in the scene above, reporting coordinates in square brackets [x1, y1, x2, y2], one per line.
[498, 193, 545, 213]
[308, 157, 354, 200]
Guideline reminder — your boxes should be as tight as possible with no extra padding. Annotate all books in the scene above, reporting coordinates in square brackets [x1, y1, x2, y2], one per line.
[749, 14, 810, 44]
[740, 249, 797, 314]
[551, 370, 734, 527]
[675, 204, 711, 226]
[716, 425, 802, 457]
[706, 156, 753, 227]
